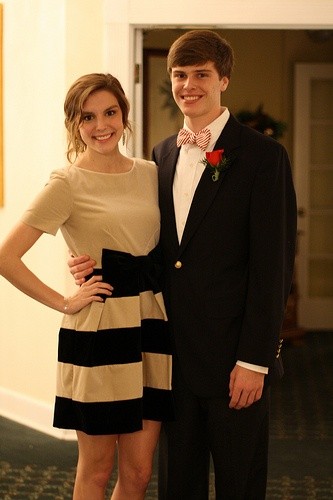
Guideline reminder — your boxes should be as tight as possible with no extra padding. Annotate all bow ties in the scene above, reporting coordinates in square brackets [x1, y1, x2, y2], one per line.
[175, 127, 213, 150]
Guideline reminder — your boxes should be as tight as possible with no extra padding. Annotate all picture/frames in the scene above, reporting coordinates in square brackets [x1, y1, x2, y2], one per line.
[143, 47, 184, 162]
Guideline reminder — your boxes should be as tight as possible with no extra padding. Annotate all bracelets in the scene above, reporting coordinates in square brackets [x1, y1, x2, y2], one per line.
[64, 296, 68, 314]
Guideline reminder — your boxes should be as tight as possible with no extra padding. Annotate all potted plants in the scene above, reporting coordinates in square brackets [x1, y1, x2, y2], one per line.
[280, 206, 306, 348]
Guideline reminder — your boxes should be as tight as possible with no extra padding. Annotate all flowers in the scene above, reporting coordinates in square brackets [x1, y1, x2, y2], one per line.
[199, 148, 229, 182]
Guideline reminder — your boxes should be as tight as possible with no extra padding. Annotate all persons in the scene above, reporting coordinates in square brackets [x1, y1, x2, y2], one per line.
[1, 74, 176, 500]
[68, 31, 297, 500]
